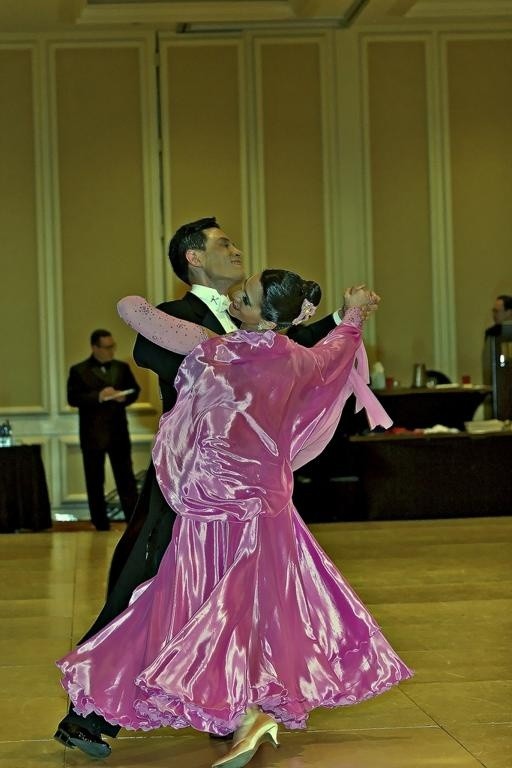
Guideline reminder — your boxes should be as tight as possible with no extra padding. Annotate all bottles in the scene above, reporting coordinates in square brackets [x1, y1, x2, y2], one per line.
[370, 361, 387, 390]
[0, 419, 13, 448]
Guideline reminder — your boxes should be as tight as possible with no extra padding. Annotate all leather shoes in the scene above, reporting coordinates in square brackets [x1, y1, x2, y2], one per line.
[51, 719, 112, 759]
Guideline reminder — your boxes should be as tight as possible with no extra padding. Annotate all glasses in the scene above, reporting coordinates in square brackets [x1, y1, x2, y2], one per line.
[97, 343, 116, 350]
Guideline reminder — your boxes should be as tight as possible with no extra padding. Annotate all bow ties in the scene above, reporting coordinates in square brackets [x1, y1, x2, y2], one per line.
[218, 294, 235, 317]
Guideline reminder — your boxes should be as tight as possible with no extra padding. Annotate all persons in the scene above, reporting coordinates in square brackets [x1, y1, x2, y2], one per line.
[66, 330, 140, 532]
[484, 295, 512, 341]
[55, 268, 415, 768]
[54, 217, 381, 758]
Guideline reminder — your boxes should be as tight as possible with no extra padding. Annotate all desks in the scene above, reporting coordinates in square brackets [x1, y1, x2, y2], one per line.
[1, 443, 52, 534]
[322, 383, 508, 518]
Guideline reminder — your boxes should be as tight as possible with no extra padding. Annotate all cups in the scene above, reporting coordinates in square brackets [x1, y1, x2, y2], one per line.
[415, 363, 426, 388]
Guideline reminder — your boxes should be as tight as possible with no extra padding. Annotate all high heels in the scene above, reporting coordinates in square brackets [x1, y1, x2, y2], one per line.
[210, 711, 281, 768]
